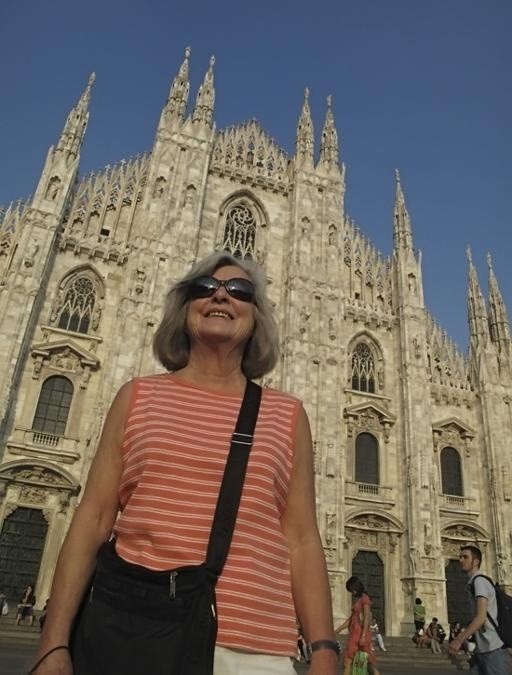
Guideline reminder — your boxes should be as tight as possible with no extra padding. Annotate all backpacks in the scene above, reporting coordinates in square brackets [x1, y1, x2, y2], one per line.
[470, 574, 512, 649]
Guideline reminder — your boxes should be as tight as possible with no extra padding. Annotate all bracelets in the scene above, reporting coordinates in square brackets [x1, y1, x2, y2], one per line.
[28, 646, 69, 675]
[305, 640, 342, 658]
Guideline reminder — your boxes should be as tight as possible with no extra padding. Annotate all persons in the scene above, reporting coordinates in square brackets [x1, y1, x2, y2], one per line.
[32, 251, 342, 675]
[295, 634, 308, 663]
[413, 598, 426, 648]
[370, 617, 387, 652]
[39, 598, 50, 633]
[16, 585, 36, 625]
[449, 545, 512, 675]
[413, 616, 469, 656]
[335, 575, 381, 675]
[0, 590, 7, 616]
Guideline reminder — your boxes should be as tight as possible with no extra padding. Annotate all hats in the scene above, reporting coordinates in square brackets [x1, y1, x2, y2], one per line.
[183, 275, 260, 308]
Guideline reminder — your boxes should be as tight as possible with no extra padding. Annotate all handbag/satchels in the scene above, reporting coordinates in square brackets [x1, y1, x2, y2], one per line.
[351, 650, 370, 675]
[66, 547, 221, 675]
